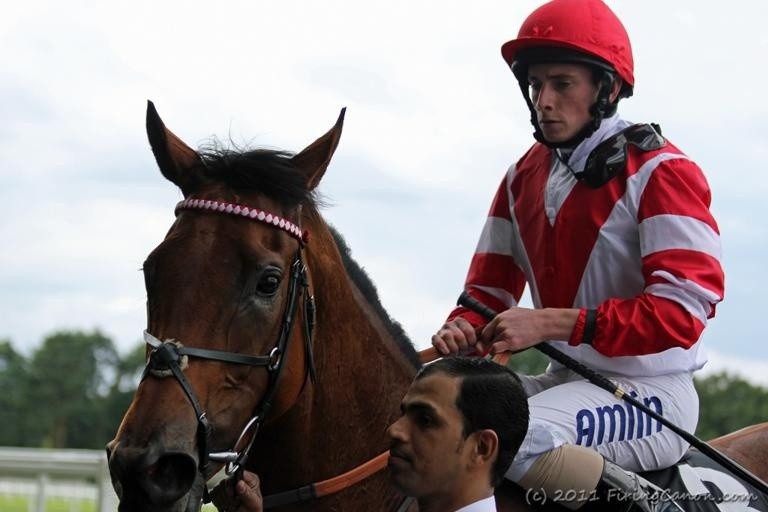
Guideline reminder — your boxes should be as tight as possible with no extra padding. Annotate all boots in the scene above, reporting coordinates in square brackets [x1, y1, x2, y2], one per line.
[570, 453, 686, 512]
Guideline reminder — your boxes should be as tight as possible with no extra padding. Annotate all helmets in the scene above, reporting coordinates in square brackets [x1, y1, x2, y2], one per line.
[502, 1, 634, 88]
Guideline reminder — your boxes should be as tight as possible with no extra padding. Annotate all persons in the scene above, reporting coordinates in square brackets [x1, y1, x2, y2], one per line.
[431, 1, 724, 512]
[212, 355, 529, 512]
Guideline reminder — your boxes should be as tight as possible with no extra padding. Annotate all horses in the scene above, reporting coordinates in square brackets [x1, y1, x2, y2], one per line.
[100, 96, 768, 512]
